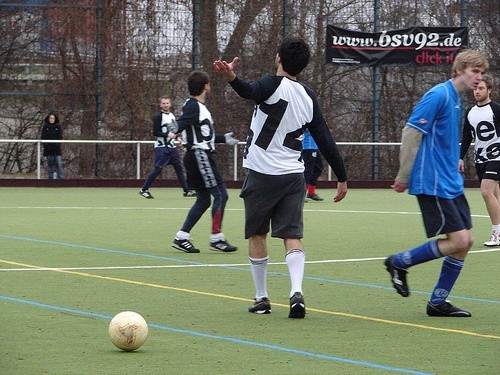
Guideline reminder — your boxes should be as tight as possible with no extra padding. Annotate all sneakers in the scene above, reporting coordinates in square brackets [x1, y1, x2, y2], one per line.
[139, 190, 154, 198]
[385, 255, 409, 296]
[183, 192, 196, 196]
[210, 241, 237, 253]
[172, 239, 200, 253]
[307, 193, 324, 200]
[427, 300, 472, 317]
[289, 292, 305, 318]
[249, 297, 271, 314]
[484, 230, 500, 246]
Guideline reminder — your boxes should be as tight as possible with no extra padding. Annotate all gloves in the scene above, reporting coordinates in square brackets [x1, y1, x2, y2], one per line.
[225, 132, 239, 146]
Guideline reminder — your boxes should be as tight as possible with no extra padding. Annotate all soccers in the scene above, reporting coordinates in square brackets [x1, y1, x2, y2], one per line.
[108, 311, 148, 351]
